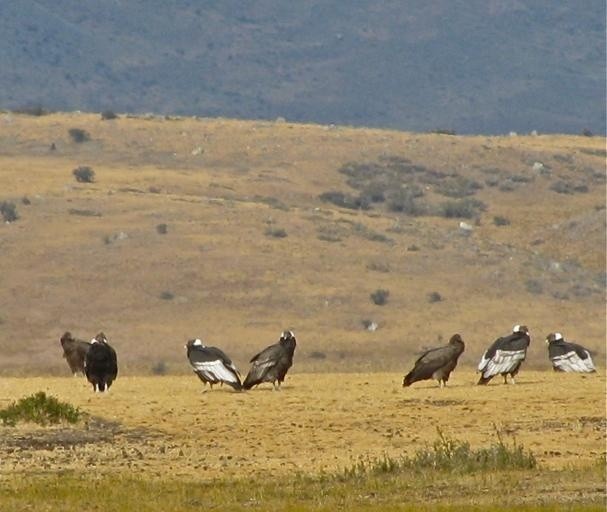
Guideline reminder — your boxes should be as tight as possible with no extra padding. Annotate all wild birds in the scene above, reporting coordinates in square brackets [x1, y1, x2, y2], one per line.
[83, 332, 118, 392]
[183, 338, 242, 394]
[401, 334, 465, 388]
[476, 324, 531, 386]
[545, 332, 596, 374]
[60, 332, 91, 382]
[242, 330, 297, 392]
